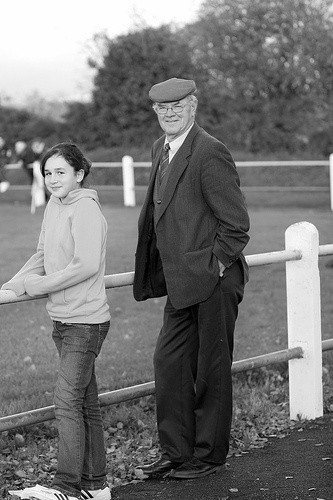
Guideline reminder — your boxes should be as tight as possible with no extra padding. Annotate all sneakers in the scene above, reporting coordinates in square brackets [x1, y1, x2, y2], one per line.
[80, 486, 112, 500]
[30, 487, 82, 500]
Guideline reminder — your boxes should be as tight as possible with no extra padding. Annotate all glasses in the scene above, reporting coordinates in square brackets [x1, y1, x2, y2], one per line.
[153, 99, 192, 114]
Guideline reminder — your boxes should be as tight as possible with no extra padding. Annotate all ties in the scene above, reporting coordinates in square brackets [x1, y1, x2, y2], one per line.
[156, 143, 171, 188]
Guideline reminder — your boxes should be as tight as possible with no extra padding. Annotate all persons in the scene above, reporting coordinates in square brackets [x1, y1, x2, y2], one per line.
[1, 143, 111, 499]
[132, 77, 250, 478]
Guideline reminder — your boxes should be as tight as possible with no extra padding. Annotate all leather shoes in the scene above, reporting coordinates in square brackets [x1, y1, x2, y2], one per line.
[169, 459, 222, 478]
[135, 455, 184, 475]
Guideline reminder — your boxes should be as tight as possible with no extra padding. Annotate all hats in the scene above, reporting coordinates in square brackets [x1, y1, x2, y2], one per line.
[148, 76, 197, 104]
